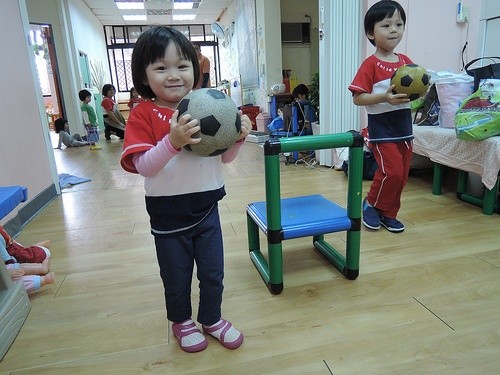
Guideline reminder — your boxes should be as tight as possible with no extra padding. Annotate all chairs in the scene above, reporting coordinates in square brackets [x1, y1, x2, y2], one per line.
[246, 130, 364, 295]
[271, 100, 316, 161]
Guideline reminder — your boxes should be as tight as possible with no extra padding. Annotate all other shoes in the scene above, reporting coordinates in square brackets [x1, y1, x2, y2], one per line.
[171, 318, 208, 353]
[201, 318, 243, 350]
[105, 129, 111, 140]
[90, 145, 102, 150]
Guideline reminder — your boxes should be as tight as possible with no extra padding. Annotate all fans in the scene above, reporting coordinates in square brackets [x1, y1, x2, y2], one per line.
[211, 21, 229, 48]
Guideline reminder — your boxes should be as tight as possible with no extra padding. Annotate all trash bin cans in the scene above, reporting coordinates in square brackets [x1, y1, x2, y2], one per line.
[255, 112, 272, 133]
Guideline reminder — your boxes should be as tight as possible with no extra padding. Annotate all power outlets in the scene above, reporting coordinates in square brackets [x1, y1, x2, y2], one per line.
[456, 2, 471, 24]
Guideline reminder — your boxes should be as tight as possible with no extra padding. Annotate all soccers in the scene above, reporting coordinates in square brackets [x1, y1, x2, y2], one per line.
[390, 64, 429, 101]
[176, 88, 242, 157]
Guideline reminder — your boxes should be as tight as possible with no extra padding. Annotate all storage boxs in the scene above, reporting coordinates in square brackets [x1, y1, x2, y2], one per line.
[284, 79, 299, 93]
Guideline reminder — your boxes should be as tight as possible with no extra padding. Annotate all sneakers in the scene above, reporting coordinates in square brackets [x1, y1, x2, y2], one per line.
[362, 197, 381, 229]
[379, 215, 405, 232]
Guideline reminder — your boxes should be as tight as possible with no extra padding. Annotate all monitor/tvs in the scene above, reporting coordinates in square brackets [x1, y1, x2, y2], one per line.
[281, 22, 310, 44]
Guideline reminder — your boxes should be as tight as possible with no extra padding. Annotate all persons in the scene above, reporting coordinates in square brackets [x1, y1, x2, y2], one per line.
[0, 225, 55, 293]
[348, 0, 432, 233]
[277, 84, 317, 164]
[119, 26, 252, 353]
[127, 87, 143, 115]
[193, 42, 210, 91]
[101, 84, 126, 141]
[79, 89, 103, 150]
[54, 117, 88, 149]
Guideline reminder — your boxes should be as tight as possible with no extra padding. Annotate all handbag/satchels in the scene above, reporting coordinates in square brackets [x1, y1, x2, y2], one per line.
[464, 56, 500, 93]
